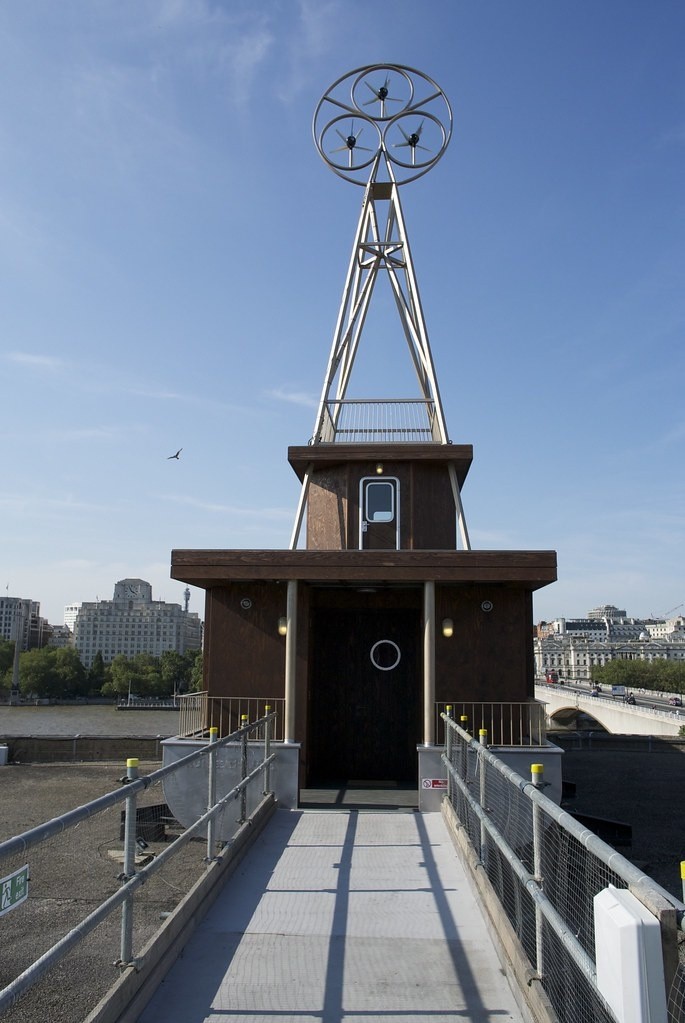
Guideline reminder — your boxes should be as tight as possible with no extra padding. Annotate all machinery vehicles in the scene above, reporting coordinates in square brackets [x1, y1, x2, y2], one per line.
[624, 691, 636, 705]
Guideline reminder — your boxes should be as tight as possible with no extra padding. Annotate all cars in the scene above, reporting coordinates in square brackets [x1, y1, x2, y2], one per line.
[589, 690, 598, 697]
[669, 697, 682, 706]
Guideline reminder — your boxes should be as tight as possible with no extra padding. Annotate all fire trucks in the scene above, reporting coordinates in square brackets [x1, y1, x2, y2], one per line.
[545, 672, 558, 684]
